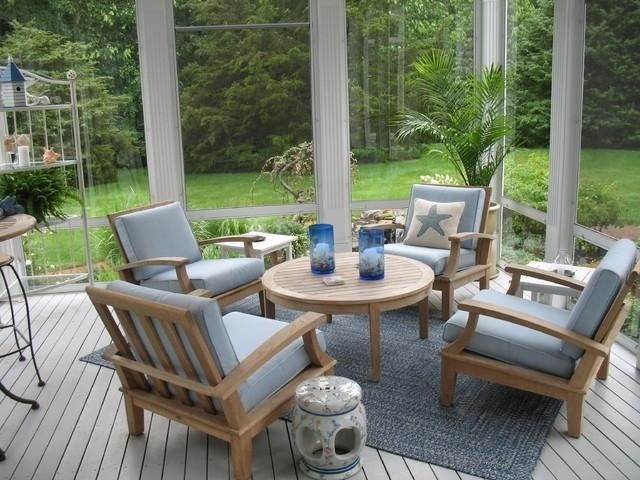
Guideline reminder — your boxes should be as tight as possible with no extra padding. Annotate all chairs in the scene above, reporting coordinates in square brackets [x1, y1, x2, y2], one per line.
[368, 185, 493, 321]
[106, 201, 265, 318]
[85, 280, 337, 480]
[440, 238, 640, 438]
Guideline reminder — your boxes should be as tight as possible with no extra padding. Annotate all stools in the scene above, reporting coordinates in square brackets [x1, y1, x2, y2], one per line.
[291, 376, 366, 480]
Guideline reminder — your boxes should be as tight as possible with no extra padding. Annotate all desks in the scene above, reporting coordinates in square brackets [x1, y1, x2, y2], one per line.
[262, 252, 435, 380]
[0, 208, 47, 410]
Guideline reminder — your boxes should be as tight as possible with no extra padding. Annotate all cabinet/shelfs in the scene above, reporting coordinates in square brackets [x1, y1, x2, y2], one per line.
[0, 58, 91, 300]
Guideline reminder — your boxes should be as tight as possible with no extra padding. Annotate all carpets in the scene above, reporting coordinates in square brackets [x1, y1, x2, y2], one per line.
[77, 289, 568, 480]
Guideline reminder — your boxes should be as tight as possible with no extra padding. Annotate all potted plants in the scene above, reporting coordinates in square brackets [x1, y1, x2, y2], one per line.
[389, 50, 529, 279]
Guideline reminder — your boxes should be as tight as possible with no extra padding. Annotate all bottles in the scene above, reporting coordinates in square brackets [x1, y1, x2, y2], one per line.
[18, 146, 30, 166]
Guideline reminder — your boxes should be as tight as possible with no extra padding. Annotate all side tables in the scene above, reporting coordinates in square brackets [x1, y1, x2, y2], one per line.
[214, 231, 298, 263]
[508, 261, 595, 310]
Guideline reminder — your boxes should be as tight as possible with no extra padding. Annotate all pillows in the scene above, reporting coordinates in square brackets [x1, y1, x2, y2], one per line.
[403, 197, 466, 251]
